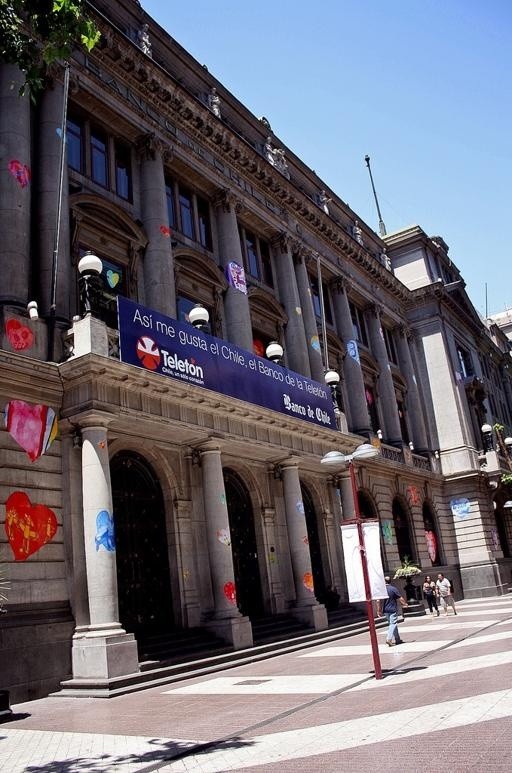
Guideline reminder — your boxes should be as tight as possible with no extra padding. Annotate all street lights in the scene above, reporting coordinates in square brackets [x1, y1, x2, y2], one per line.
[320, 444, 383, 679]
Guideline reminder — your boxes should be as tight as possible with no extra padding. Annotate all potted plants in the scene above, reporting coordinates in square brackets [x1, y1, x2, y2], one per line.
[393, 556, 422, 604]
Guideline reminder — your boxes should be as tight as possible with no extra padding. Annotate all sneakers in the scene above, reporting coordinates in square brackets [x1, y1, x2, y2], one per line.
[386, 639, 396, 645]
[396, 637, 403, 642]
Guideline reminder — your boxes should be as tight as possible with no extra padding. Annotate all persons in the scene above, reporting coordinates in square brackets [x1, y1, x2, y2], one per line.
[422, 574, 440, 617]
[376, 576, 410, 645]
[434, 571, 457, 616]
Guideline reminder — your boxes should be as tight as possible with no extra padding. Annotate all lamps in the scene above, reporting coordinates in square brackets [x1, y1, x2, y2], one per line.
[266, 340, 284, 364]
[324, 369, 340, 411]
[187, 304, 210, 331]
[78, 251, 103, 317]
[481, 424, 512, 457]
